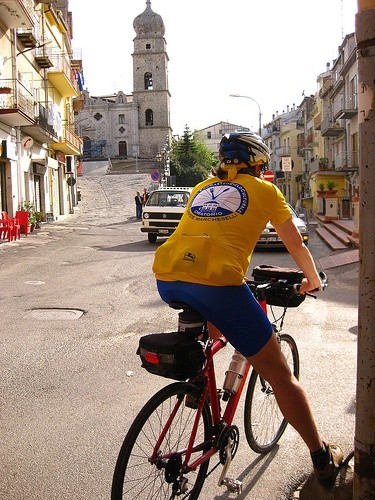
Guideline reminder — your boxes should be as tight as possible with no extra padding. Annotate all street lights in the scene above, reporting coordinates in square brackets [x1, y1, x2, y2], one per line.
[155, 143, 168, 187]
[230, 95, 261, 137]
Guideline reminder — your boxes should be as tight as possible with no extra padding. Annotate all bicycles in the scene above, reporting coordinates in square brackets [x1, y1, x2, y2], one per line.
[111, 264, 327, 500]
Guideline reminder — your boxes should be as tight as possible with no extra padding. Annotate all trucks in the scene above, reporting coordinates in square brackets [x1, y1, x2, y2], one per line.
[141, 189, 191, 243]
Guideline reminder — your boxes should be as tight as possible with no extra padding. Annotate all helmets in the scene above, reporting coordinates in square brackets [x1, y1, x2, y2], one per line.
[219, 131, 270, 165]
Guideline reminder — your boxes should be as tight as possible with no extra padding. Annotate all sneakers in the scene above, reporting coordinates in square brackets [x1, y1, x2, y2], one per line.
[185, 376, 222, 410]
[312, 441, 344, 486]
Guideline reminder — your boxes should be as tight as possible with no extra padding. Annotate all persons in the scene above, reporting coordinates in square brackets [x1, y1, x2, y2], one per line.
[134, 188, 150, 219]
[153, 130, 345, 486]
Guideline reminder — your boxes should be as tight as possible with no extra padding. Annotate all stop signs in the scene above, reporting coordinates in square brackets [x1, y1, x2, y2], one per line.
[264, 171, 274, 182]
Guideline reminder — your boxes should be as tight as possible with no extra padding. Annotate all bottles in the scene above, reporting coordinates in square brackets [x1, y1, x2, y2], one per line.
[223, 349, 249, 394]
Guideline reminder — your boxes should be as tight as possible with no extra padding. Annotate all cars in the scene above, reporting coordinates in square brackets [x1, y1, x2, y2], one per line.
[254, 203, 309, 252]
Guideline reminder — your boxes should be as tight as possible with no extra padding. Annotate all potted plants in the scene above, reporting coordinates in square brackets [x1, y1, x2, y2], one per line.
[24, 201, 37, 234]
[327, 182, 335, 191]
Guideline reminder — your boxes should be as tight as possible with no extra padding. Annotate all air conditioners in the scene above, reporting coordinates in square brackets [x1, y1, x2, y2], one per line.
[63, 154, 75, 173]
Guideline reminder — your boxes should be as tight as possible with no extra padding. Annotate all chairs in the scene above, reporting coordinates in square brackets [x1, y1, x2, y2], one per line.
[0, 211, 29, 242]
